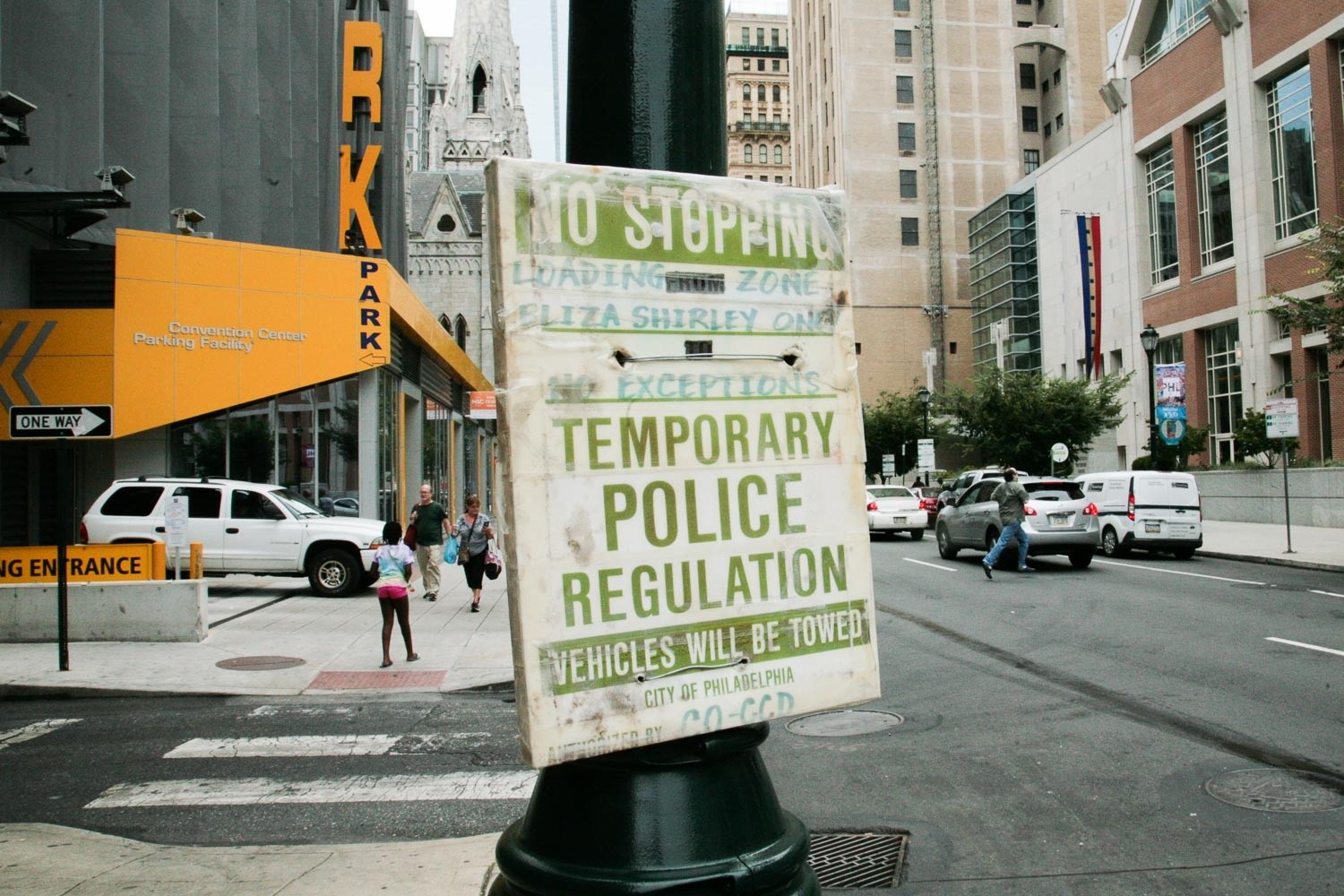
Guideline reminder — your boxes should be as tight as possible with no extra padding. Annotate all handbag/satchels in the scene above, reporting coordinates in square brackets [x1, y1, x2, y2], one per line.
[403, 524, 416, 551]
[484, 543, 502, 581]
[444, 534, 457, 565]
[458, 542, 470, 565]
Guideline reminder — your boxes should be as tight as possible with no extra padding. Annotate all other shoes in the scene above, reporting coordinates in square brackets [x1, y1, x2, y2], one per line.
[1020, 566, 1036, 572]
[983, 563, 992, 579]
[471, 597, 479, 612]
[423, 593, 430, 598]
[429, 594, 436, 601]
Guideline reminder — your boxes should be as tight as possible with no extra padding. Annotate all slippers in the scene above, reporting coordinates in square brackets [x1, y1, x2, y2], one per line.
[407, 653, 420, 662]
[380, 661, 392, 667]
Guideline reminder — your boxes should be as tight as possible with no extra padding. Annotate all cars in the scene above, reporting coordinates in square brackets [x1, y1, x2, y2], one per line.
[935, 475, 1101, 568]
[909, 487, 944, 529]
[333, 497, 359, 517]
[865, 485, 928, 541]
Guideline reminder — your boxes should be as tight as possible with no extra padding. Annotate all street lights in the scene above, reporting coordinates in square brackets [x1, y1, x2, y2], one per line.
[1139, 322, 1160, 470]
[918, 386, 930, 486]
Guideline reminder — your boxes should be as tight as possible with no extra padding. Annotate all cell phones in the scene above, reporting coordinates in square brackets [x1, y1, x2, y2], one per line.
[483, 521, 490, 528]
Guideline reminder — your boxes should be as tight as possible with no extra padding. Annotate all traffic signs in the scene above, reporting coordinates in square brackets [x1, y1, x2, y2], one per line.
[8, 404, 114, 439]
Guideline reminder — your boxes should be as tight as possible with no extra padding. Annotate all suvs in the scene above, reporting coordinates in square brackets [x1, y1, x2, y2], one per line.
[935, 468, 1030, 518]
[79, 474, 389, 598]
[1073, 469, 1203, 561]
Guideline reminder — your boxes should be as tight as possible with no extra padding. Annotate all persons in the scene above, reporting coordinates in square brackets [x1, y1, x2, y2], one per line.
[981, 467, 1035, 579]
[368, 521, 420, 667]
[937, 477, 947, 488]
[451, 494, 493, 611]
[410, 484, 452, 601]
[912, 476, 924, 488]
[318, 488, 334, 512]
